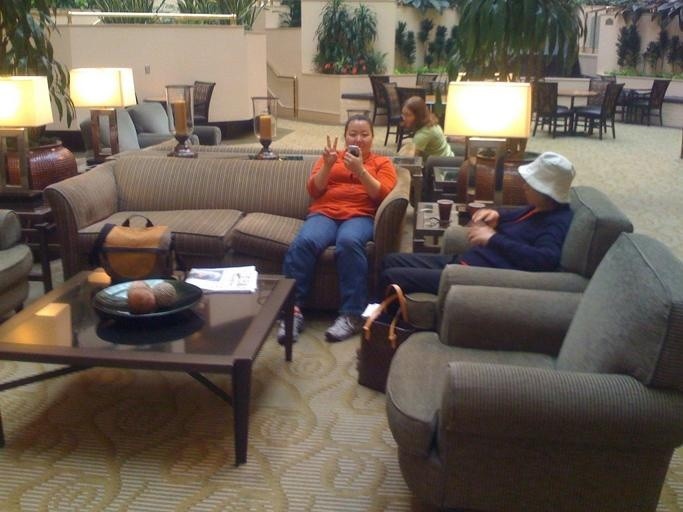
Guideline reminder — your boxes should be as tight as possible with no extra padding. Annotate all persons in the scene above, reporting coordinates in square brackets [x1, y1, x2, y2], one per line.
[277, 115, 396, 346]
[400, 96, 456, 159]
[360, 150, 578, 317]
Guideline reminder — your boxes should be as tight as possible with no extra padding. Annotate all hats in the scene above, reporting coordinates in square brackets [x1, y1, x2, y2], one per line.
[515, 150, 574, 207]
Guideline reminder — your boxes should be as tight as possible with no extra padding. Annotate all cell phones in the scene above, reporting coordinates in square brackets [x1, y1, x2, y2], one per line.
[348, 145, 359, 157]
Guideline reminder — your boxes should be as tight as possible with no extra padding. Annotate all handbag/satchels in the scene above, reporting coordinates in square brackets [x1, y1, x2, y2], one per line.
[355, 283, 419, 395]
[89, 214, 180, 277]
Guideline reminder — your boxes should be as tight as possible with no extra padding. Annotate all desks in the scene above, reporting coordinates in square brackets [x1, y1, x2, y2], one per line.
[432, 163, 480, 198]
[413, 199, 498, 250]
[5, 196, 59, 313]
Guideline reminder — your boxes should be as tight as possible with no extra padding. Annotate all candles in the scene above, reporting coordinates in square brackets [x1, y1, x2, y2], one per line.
[259, 114, 271, 139]
[173, 100, 188, 133]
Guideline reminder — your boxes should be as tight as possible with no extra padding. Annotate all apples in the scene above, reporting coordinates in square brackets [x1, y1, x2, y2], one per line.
[127, 280, 151, 302]
[129, 289, 154, 315]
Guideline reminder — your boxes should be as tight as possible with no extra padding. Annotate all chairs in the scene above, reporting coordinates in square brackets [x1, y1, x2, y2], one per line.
[80, 108, 137, 152]
[123, 100, 221, 149]
[4, 144, 87, 244]
[341, 73, 448, 154]
[532, 72, 671, 141]
[398, 186, 635, 332]
[0, 210, 33, 326]
[421, 140, 526, 197]
[186, 82, 216, 126]
[381, 232, 683, 511]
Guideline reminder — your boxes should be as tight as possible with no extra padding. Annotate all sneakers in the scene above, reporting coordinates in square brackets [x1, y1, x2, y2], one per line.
[277, 311, 306, 345]
[325, 315, 365, 341]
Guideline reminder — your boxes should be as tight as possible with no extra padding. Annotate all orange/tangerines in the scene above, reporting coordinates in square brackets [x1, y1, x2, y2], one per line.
[153, 283, 177, 307]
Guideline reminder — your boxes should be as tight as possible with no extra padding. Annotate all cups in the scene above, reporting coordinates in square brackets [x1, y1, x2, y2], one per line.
[436, 198, 452, 221]
[468, 202, 485, 216]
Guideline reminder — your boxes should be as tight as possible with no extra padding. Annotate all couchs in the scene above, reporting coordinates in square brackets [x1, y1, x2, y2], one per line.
[44, 147, 410, 321]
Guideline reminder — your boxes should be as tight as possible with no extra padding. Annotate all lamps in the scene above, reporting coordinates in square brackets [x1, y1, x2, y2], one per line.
[67, 67, 136, 166]
[0, 74, 54, 207]
[442, 78, 532, 203]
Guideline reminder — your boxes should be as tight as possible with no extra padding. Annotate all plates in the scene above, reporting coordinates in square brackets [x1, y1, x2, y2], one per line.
[96, 307, 205, 346]
[92, 279, 204, 319]
